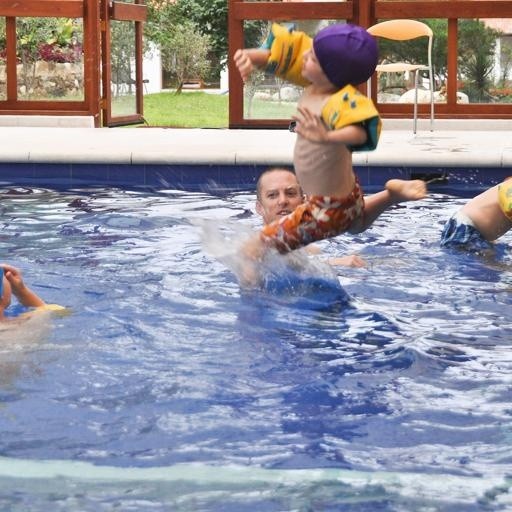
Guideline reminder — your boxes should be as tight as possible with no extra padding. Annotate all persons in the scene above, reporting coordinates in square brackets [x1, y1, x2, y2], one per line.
[236, 166, 423, 376]
[441, 173, 512, 280]
[233, 16, 432, 293]
[0, 263, 70, 402]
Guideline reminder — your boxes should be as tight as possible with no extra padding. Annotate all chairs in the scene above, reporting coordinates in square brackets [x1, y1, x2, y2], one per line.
[364, 18, 435, 134]
[109, 64, 150, 98]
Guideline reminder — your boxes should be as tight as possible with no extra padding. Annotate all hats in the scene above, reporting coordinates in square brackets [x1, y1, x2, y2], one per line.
[313, 23, 377, 88]
